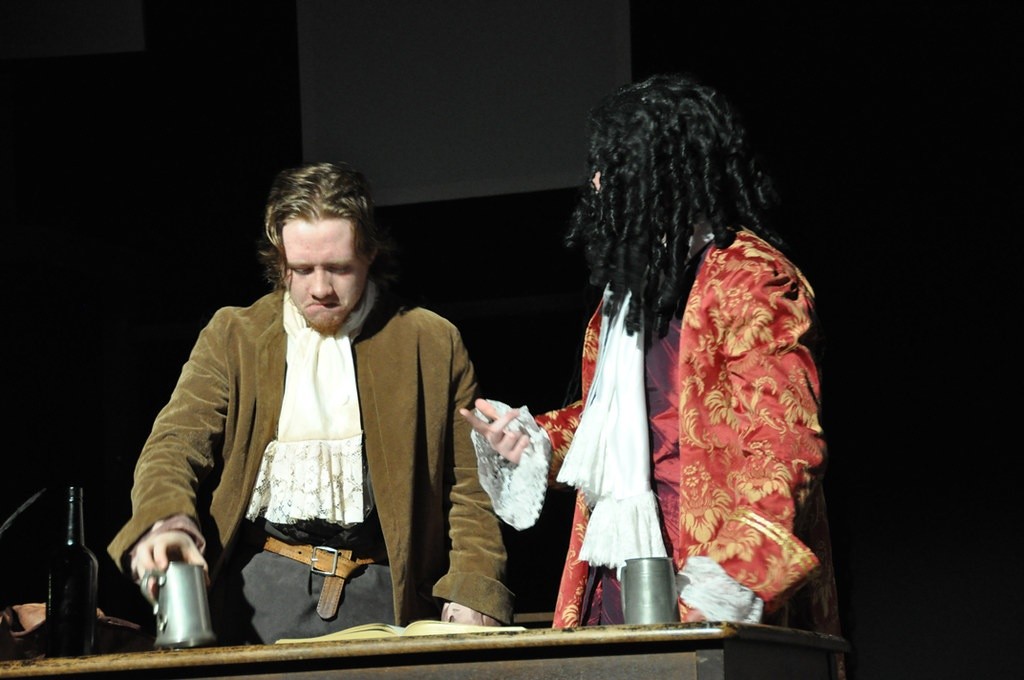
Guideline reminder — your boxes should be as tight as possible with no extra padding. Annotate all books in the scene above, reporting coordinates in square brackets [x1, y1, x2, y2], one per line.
[274, 618, 525, 646]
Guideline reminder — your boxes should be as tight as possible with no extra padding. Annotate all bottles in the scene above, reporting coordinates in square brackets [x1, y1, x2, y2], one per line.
[44, 486, 99, 658]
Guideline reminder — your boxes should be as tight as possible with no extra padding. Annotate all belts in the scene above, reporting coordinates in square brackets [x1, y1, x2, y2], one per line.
[257, 532, 389, 619]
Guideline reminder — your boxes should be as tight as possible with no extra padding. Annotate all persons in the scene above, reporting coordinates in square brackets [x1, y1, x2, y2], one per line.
[108, 160, 515, 647]
[459, 61, 847, 680]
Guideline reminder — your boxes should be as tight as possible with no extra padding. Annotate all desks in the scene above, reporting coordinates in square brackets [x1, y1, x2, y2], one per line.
[0, 619, 851, 680]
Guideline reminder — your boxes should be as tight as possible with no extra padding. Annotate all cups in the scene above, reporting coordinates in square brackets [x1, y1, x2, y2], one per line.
[620, 557, 681, 625]
[139, 561, 216, 648]
